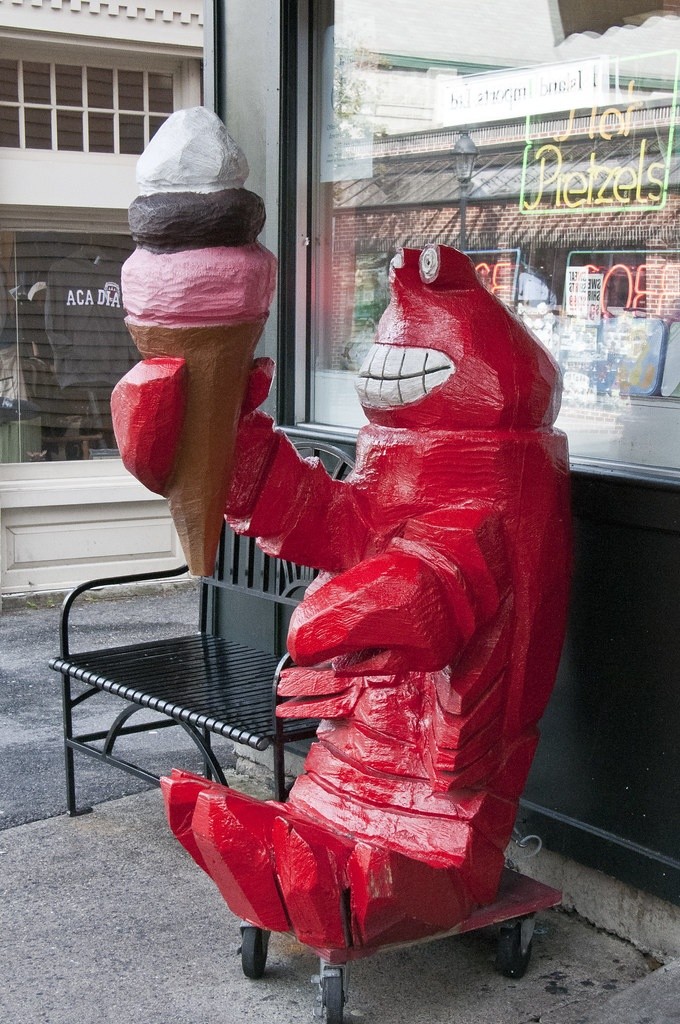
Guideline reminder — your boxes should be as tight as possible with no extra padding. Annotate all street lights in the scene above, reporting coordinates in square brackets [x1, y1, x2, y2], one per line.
[451, 129, 480, 251]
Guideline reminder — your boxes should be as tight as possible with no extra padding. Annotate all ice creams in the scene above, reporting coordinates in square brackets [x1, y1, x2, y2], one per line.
[120, 105, 278, 579]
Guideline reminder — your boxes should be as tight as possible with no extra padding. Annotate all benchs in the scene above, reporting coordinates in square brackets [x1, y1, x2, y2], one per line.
[49, 443, 356, 817]
